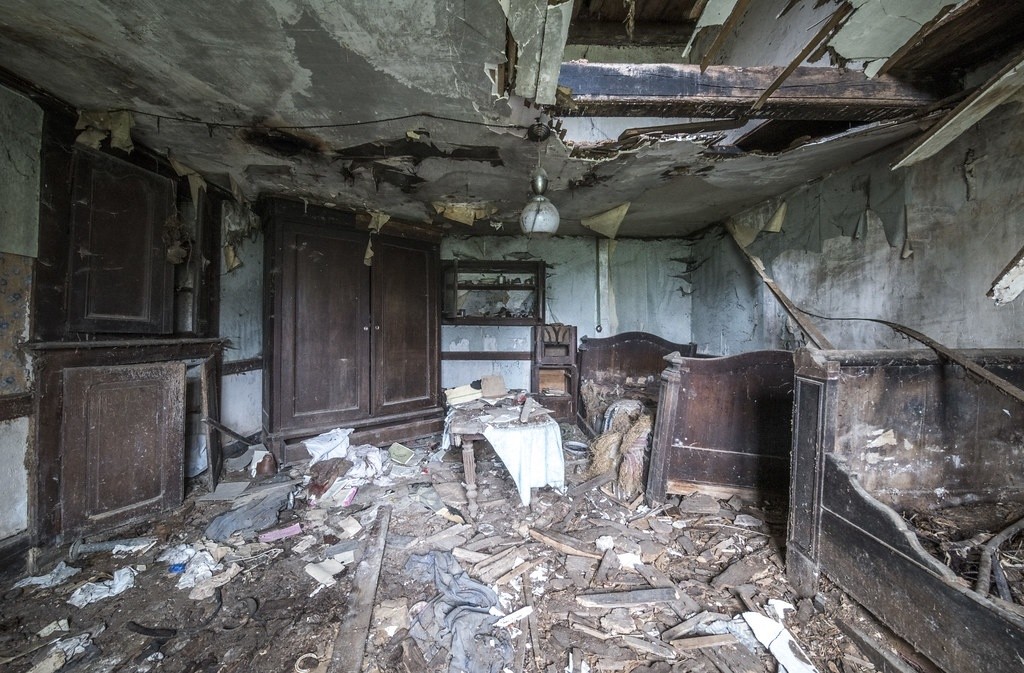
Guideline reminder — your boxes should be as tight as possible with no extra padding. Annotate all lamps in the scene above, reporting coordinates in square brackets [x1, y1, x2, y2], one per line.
[519, 123, 561, 242]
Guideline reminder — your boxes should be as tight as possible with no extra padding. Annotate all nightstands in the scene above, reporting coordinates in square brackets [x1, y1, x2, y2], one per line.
[530, 323, 578, 427]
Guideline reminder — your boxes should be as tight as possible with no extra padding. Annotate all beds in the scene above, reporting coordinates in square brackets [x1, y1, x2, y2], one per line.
[569, 332, 794, 510]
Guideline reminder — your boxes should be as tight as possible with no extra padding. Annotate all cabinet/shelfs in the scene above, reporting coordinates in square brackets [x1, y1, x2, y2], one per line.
[26, 339, 225, 564]
[251, 191, 446, 472]
[32, 101, 207, 338]
[440, 259, 546, 327]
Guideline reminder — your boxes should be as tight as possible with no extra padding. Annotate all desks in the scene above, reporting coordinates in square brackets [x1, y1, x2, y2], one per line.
[446, 395, 557, 520]
